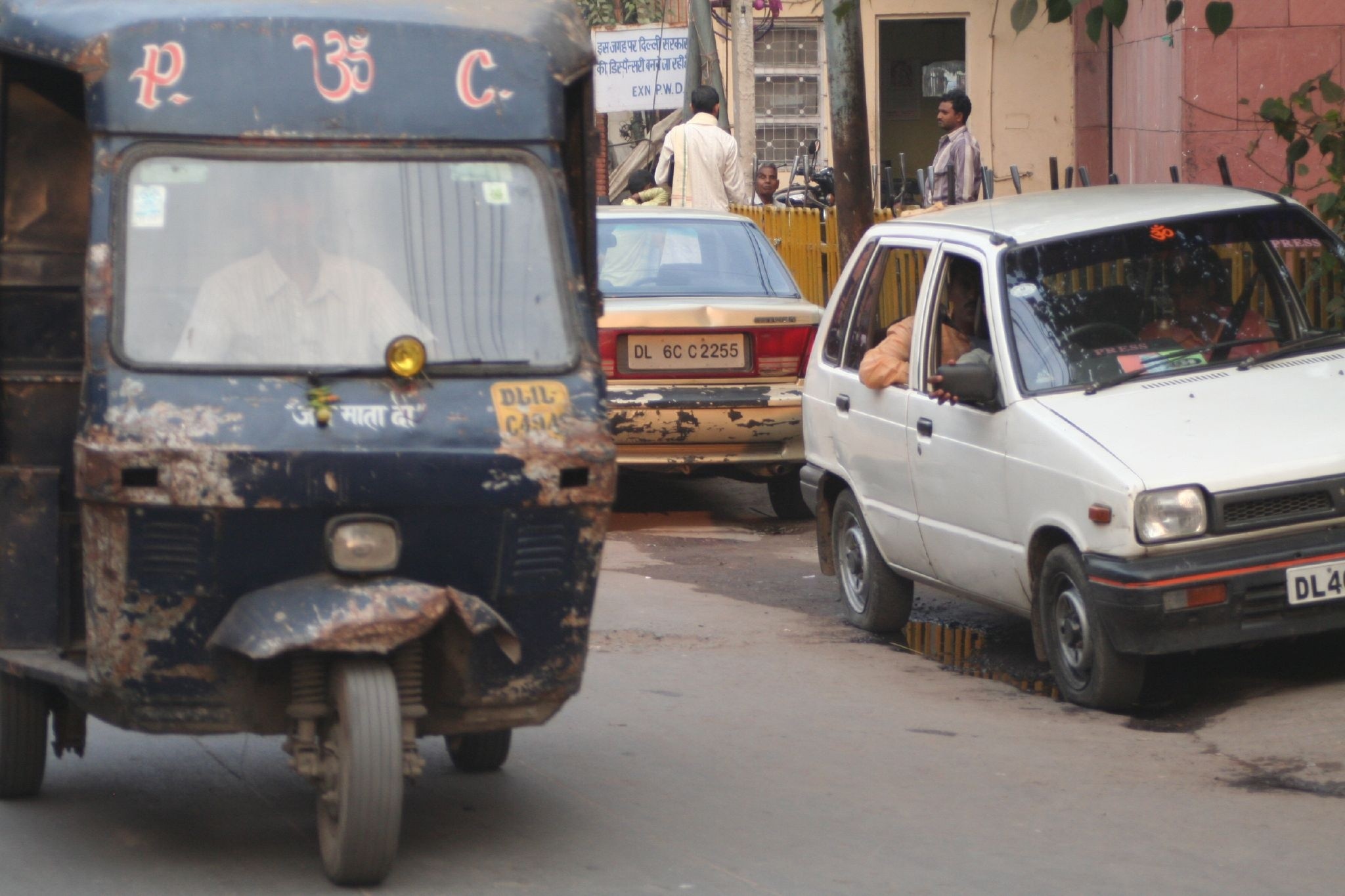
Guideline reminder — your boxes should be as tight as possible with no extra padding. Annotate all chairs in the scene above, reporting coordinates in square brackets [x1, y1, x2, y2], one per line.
[656, 263, 711, 288]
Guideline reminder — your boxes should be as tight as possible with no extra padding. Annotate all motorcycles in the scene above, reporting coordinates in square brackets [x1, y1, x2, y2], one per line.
[2, 0, 616, 887]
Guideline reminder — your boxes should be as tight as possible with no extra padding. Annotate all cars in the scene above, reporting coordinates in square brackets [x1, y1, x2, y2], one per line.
[584, 208, 830, 522]
[807, 185, 1345, 713]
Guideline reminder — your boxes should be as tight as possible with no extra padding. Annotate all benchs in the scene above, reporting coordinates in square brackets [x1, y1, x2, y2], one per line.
[1049, 285, 1155, 354]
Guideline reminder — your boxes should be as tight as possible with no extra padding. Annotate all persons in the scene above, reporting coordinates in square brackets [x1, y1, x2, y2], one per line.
[170, 174, 436, 365]
[858, 256, 1072, 411]
[748, 162, 791, 226]
[926, 88, 982, 204]
[1136, 238, 1279, 363]
[599, 170, 669, 289]
[1140, 354, 1202, 373]
[654, 86, 748, 286]
